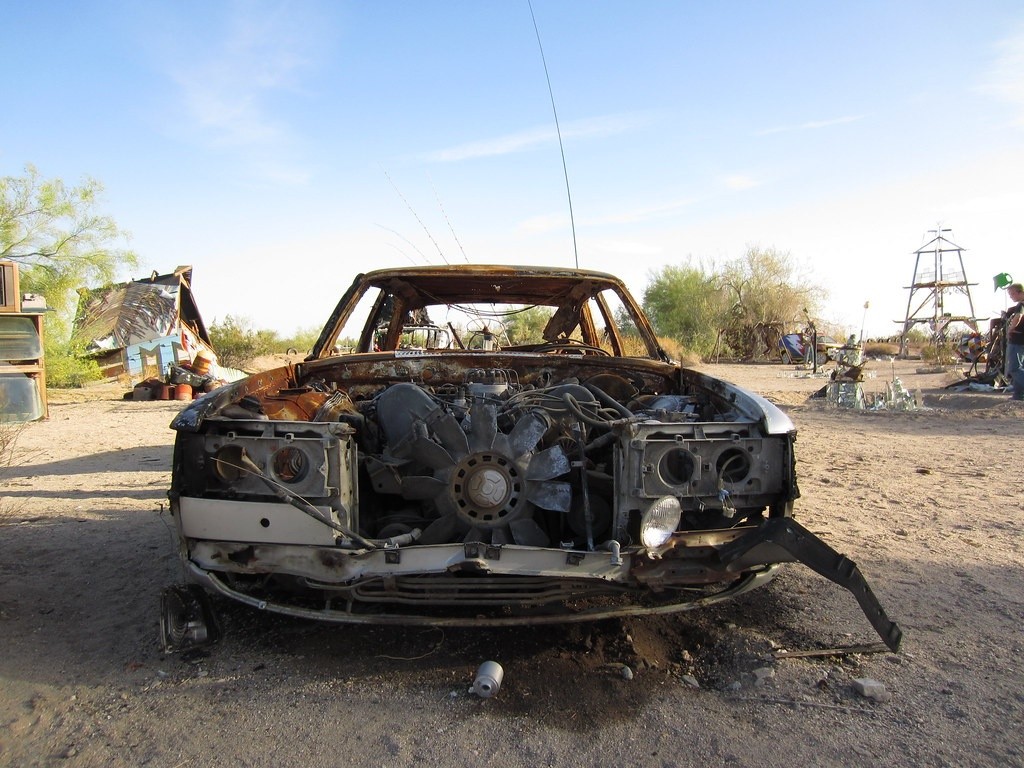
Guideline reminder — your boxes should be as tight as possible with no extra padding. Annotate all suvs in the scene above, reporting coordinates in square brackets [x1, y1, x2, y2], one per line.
[779, 333, 845, 365]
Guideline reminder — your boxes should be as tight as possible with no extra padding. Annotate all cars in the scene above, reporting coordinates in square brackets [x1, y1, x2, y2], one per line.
[169, 266, 903, 655]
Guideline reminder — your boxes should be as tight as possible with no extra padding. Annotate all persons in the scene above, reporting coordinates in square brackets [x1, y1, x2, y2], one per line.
[999, 284, 1024, 401]
